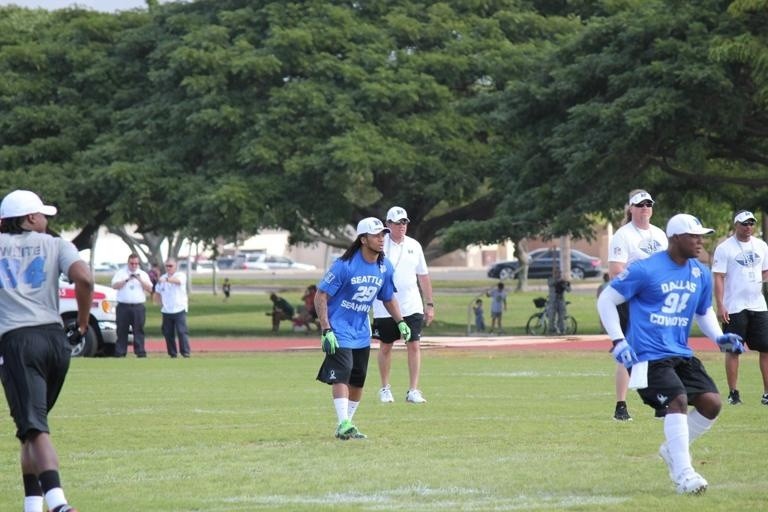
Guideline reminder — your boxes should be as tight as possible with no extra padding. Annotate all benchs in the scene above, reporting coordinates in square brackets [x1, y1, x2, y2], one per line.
[291, 304, 320, 334]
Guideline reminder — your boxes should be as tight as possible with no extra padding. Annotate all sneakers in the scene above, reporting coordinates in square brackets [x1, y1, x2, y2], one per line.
[614, 404, 632, 423]
[334, 421, 367, 441]
[654, 407, 667, 417]
[659, 443, 709, 495]
[406, 388, 426, 404]
[379, 383, 394, 403]
[727, 389, 743, 404]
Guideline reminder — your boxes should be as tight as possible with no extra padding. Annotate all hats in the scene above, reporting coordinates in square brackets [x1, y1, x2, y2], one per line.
[733, 212, 757, 226]
[667, 214, 715, 238]
[629, 191, 655, 207]
[357, 216, 391, 236]
[386, 206, 410, 223]
[0, 190, 57, 219]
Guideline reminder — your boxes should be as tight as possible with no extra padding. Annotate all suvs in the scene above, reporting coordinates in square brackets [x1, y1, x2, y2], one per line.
[57, 272, 134, 355]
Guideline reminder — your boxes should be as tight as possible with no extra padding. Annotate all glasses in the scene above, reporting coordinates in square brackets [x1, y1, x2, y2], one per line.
[635, 202, 653, 208]
[394, 219, 407, 225]
[741, 221, 754, 226]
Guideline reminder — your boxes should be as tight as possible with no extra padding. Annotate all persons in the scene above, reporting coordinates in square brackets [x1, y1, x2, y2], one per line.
[0, 188, 94, 512]
[598, 273, 608, 334]
[314, 217, 411, 439]
[372, 207, 434, 403]
[608, 185, 669, 423]
[589, 212, 747, 495]
[709, 210, 768, 404]
[111, 252, 190, 359]
[486, 283, 507, 333]
[270, 294, 295, 333]
[473, 299, 485, 335]
[222, 278, 230, 300]
[292, 285, 318, 327]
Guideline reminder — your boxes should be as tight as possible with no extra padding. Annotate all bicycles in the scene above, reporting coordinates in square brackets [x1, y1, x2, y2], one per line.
[527, 295, 578, 335]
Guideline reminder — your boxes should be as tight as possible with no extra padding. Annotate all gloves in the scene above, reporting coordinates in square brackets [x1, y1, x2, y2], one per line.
[716, 334, 745, 353]
[612, 339, 638, 368]
[66, 323, 87, 344]
[397, 320, 411, 344]
[321, 329, 340, 354]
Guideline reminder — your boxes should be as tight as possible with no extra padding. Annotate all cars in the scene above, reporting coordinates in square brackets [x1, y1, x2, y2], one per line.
[488, 248, 603, 281]
[91, 245, 317, 272]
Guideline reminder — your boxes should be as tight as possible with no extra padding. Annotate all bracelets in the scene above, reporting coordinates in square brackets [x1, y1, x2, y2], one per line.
[424, 302, 434, 307]
